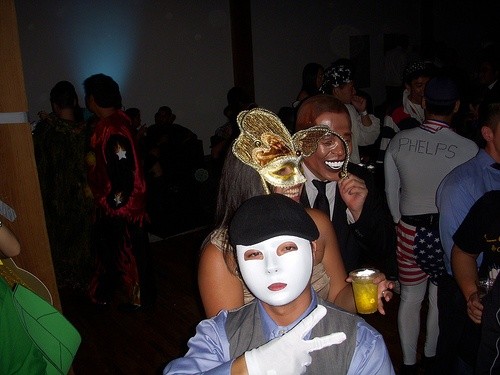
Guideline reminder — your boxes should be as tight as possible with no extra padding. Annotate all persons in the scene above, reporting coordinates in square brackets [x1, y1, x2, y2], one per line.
[318, 59, 381, 164]
[392, 60, 438, 129]
[384, 33, 411, 107]
[295, 94, 397, 274]
[33, 73, 159, 314]
[477, 57, 500, 101]
[298, 63, 324, 102]
[384, 78, 479, 375]
[126, 103, 198, 187]
[0, 217, 47, 375]
[436, 97, 500, 375]
[199, 108, 396, 319]
[163, 194, 395, 375]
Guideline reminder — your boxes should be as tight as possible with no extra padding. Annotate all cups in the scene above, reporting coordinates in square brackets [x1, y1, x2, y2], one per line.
[349, 267, 380, 314]
[475, 278, 495, 304]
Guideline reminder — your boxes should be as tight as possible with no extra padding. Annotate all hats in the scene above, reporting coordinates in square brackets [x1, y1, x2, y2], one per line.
[425, 72, 474, 108]
[226, 193, 320, 246]
[403, 61, 436, 80]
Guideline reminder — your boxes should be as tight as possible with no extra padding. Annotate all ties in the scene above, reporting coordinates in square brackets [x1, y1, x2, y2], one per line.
[311, 178, 331, 221]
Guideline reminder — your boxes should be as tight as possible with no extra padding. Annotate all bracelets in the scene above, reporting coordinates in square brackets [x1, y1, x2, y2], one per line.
[360, 110, 367, 115]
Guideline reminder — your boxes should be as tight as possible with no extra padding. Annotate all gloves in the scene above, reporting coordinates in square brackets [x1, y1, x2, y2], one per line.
[244, 304, 348, 375]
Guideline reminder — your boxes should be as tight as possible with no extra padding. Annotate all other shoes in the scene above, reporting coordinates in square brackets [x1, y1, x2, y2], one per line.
[396, 363, 419, 375]
[419, 352, 443, 375]
